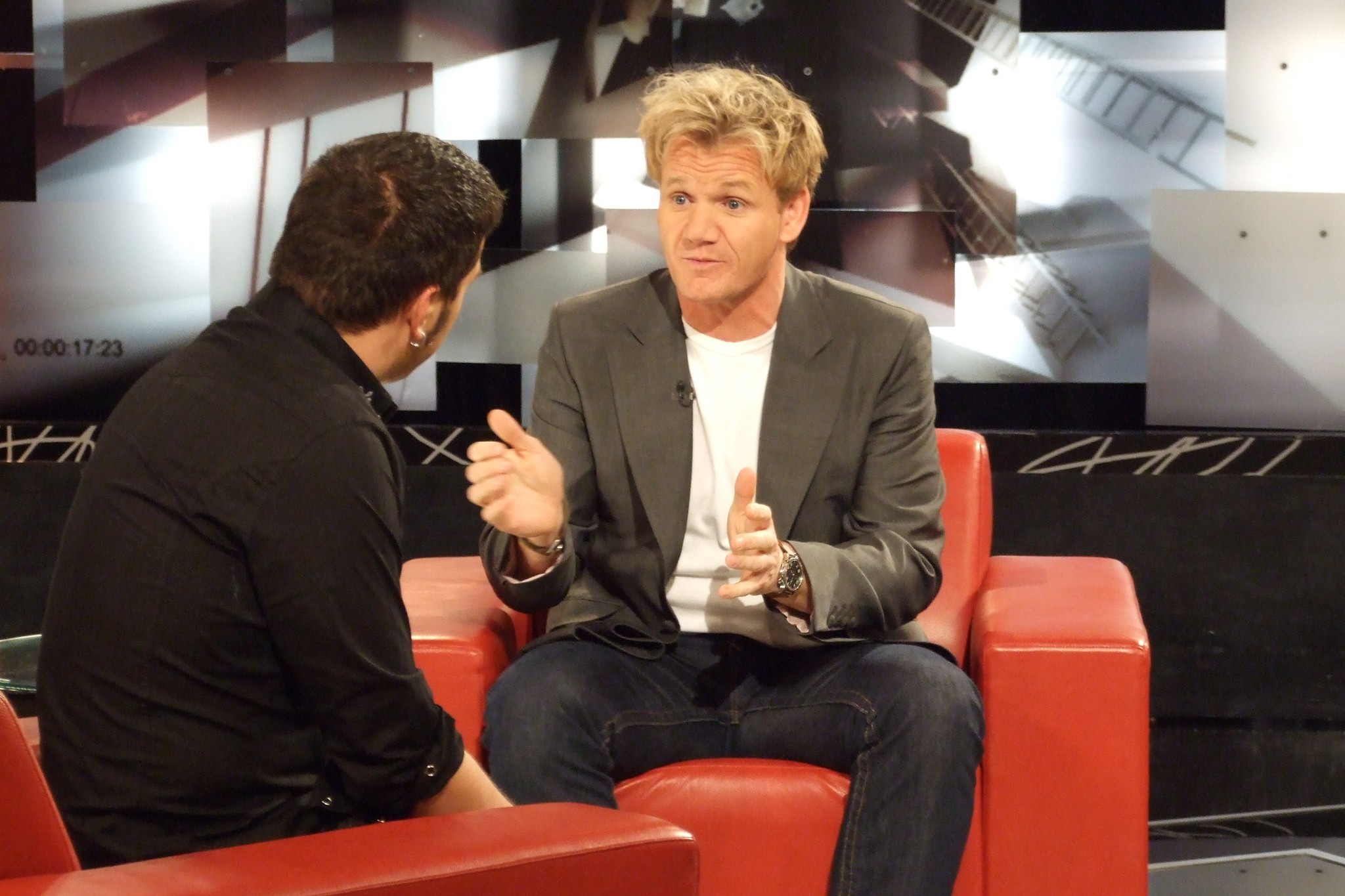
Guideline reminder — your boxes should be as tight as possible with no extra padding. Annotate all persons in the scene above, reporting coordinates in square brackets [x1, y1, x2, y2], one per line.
[37, 131, 518, 868]
[465, 62, 986, 896]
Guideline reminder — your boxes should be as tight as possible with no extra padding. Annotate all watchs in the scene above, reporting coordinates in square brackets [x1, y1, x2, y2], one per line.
[774, 540, 805, 597]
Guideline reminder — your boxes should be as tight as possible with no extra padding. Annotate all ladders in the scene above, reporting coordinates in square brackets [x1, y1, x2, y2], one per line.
[877, 108, 1107, 370]
[902, 0, 1224, 190]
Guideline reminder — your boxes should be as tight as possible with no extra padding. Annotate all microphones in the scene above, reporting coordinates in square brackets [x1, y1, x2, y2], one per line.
[677, 380, 695, 408]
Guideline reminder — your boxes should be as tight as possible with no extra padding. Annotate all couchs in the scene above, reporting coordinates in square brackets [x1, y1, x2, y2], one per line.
[398, 429, 1149, 896]
[0, 690, 703, 896]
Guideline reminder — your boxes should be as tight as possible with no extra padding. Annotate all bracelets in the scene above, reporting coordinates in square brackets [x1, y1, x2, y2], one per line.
[521, 537, 564, 556]
